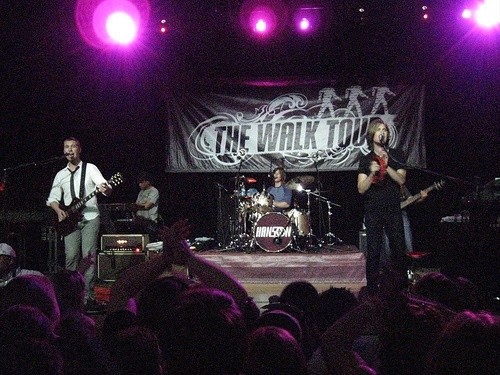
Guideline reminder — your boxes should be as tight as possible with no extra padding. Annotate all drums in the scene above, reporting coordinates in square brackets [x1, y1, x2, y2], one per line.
[283, 207, 310, 236]
[252, 211, 294, 252]
[237, 196, 252, 212]
[252, 191, 274, 212]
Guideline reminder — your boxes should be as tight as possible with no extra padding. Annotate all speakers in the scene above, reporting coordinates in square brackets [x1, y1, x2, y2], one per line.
[98, 252, 145, 282]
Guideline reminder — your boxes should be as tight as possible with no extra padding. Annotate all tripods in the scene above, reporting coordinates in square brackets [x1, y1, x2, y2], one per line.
[215, 165, 353, 252]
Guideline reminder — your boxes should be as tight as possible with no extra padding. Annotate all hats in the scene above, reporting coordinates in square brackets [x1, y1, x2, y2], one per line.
[0, 243, 16, 258]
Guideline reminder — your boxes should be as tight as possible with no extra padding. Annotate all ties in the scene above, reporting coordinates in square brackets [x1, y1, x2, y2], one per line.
[67, 166, 80, 199]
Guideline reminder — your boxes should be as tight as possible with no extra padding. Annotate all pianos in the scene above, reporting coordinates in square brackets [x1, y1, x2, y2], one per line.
[99, 202, 140, 218]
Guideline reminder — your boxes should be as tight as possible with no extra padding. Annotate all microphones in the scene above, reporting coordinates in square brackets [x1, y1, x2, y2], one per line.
[378, 134, 384, 141]
[216, 183, 229, 192]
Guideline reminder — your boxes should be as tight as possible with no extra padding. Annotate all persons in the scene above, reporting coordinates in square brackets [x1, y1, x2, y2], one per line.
[46, 137, 113, 307]
[0, 218, 500, 375]
[266, 167, 293, 209]
[132, 174, 159, 224]
[357, 118, 428, 287]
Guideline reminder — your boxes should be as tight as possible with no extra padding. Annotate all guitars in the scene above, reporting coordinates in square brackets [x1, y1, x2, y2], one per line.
[49, 172, 124, 235]
[401, 178, 448, 209]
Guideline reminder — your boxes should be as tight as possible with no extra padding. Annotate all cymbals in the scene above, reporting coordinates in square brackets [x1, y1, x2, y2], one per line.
[284, 175, 314, 189]
[230, 176, 257, 183]
